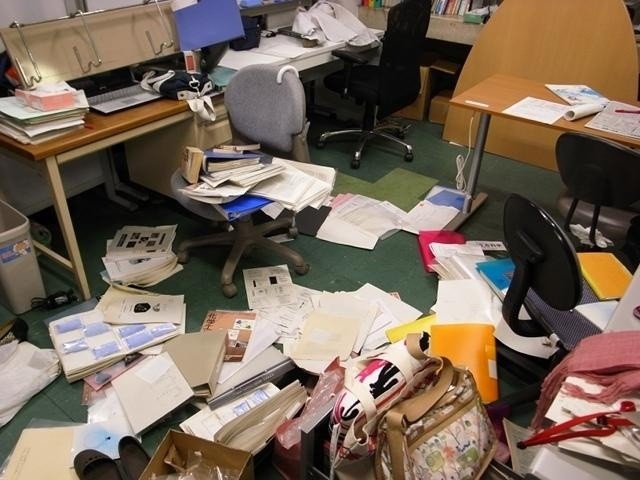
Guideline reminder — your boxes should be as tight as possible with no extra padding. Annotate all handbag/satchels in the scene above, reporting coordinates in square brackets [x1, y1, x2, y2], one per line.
[275, 333, 497, 480]
[134, 65, 213, 101]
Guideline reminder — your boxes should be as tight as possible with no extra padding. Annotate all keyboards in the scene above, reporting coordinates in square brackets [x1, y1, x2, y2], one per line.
[88, 86, 162, 112]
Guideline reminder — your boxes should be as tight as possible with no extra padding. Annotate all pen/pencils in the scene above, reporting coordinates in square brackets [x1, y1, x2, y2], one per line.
[615, 110, 640, 114]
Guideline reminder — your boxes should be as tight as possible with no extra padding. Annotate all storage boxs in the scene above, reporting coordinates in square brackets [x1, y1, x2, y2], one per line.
[391, 52, 461, 125]
[138, 428, 257, 480]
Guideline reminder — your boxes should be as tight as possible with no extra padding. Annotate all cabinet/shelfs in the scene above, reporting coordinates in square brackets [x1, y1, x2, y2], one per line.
[301, 332, 525, 480]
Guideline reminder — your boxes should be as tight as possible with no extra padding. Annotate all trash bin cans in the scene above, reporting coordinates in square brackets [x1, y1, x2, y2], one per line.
[0, 199, 48, 314]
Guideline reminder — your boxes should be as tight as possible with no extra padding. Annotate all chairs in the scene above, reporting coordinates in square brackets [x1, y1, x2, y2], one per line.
[170, 63, 312, 298]
[485, 191, 602, 418]
[555, 129, 640, 278]
[316, 0, 433, 169]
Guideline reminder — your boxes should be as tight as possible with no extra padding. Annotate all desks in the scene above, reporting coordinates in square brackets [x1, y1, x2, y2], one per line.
[436, 73, 640, 232]
[220, 27, 386, 116]
[0, 90, 231, 298]
[357, 5, 484, 46]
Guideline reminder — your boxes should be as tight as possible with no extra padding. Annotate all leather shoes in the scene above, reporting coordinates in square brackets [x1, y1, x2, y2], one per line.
[74, 450, 120, 479]
[117, 436, 151, 479]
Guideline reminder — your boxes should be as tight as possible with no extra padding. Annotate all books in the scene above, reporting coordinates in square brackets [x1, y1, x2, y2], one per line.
[363, 0, 502, 16]
[1, 83, 640, 479]
